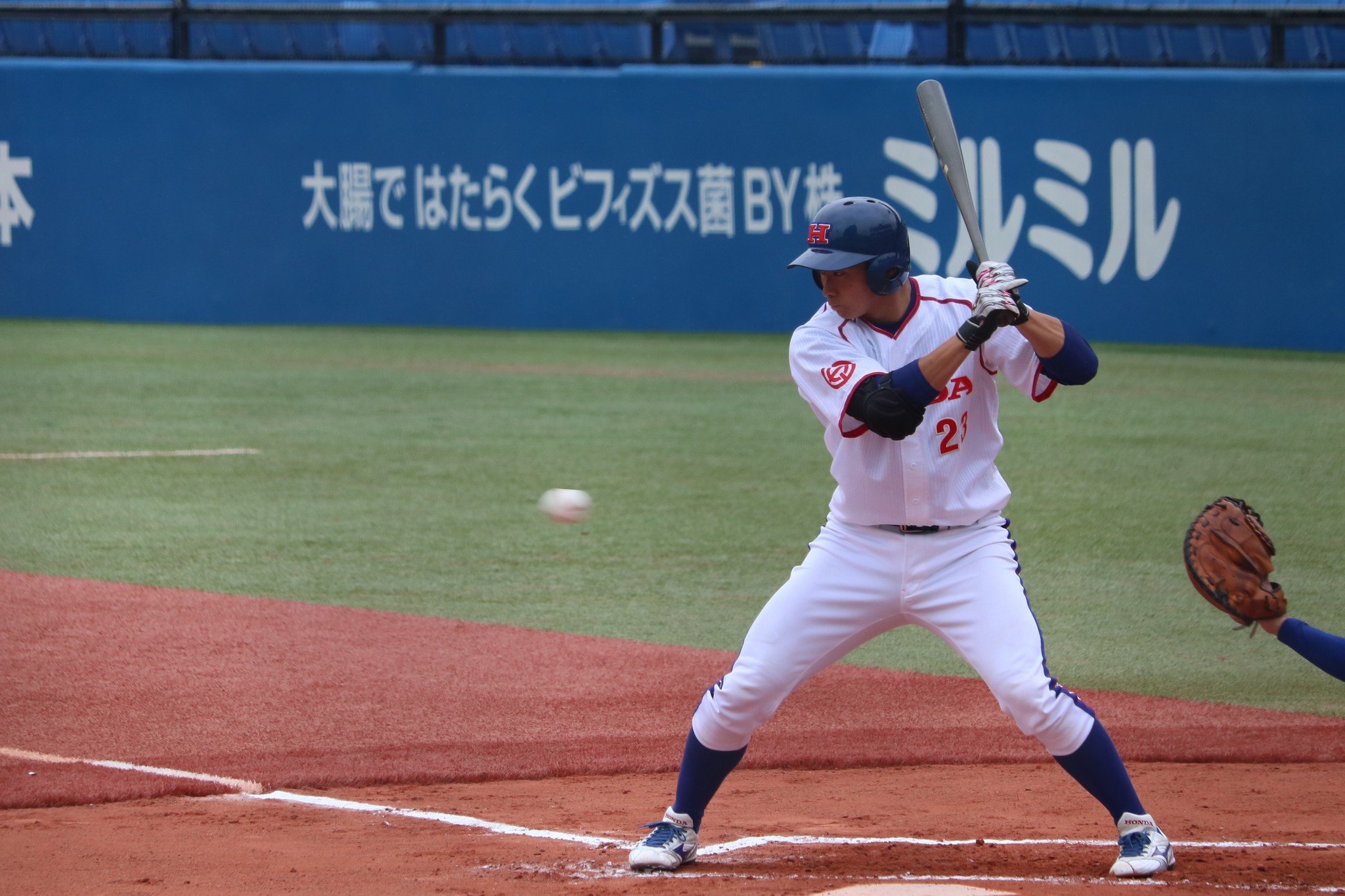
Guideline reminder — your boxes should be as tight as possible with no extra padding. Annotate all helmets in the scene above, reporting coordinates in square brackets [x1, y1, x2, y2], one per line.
[787, 195, 910, 296]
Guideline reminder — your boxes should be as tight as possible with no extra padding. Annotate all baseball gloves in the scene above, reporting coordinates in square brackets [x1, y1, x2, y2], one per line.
[1182, 495, 1290, 640]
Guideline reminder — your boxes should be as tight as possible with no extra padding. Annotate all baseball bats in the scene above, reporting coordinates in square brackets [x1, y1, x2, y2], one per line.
[914, 79, 1011, 330]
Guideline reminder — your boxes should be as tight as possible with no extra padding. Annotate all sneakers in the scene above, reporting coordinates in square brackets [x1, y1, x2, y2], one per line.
[1109, 811, 1176, 875]
[628, 805, 699, 870]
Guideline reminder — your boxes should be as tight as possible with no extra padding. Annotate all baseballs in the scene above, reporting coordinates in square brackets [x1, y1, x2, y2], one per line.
[538, 488, 591, 524]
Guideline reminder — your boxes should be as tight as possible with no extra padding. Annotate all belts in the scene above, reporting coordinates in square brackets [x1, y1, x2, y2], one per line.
[891, 523, 950, 532]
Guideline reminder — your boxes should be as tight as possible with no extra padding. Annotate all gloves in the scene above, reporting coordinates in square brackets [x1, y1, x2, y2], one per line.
[956, 286, 1019, 351]
[965, 260, 1027, 327]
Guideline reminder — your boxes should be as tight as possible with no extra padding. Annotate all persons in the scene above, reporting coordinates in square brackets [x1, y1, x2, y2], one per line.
[630, 195, 1176, 881]
[1181, 494, 1344, 694]
